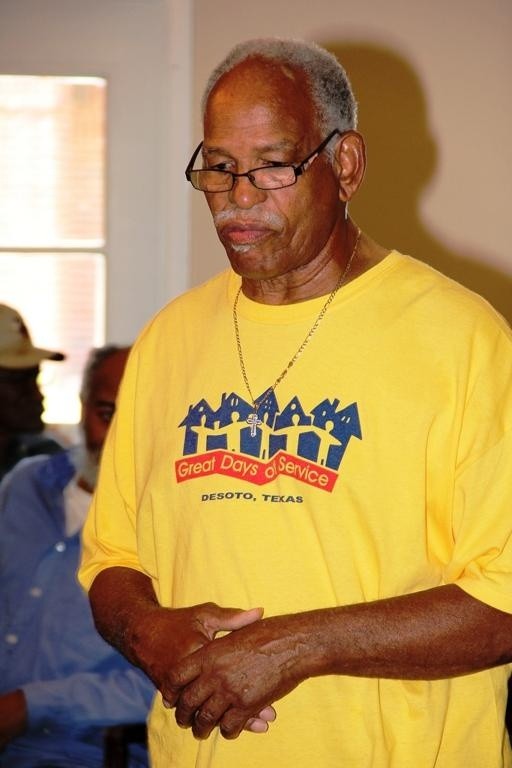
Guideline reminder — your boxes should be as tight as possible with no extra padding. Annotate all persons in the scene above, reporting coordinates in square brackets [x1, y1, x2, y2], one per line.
[0, 344, 159, 767]
[0, 302, 71, 476]
[77, 35, 512, 767]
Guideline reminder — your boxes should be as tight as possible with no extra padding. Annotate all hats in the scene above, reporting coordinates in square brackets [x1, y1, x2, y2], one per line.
[0, 302, 66, 370]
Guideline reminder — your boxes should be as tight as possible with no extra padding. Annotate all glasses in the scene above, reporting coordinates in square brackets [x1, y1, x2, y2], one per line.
[183, 128, 344, 193]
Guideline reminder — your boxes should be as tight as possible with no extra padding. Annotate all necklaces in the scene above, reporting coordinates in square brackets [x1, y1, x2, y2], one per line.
[233, 227, 363, 438]
